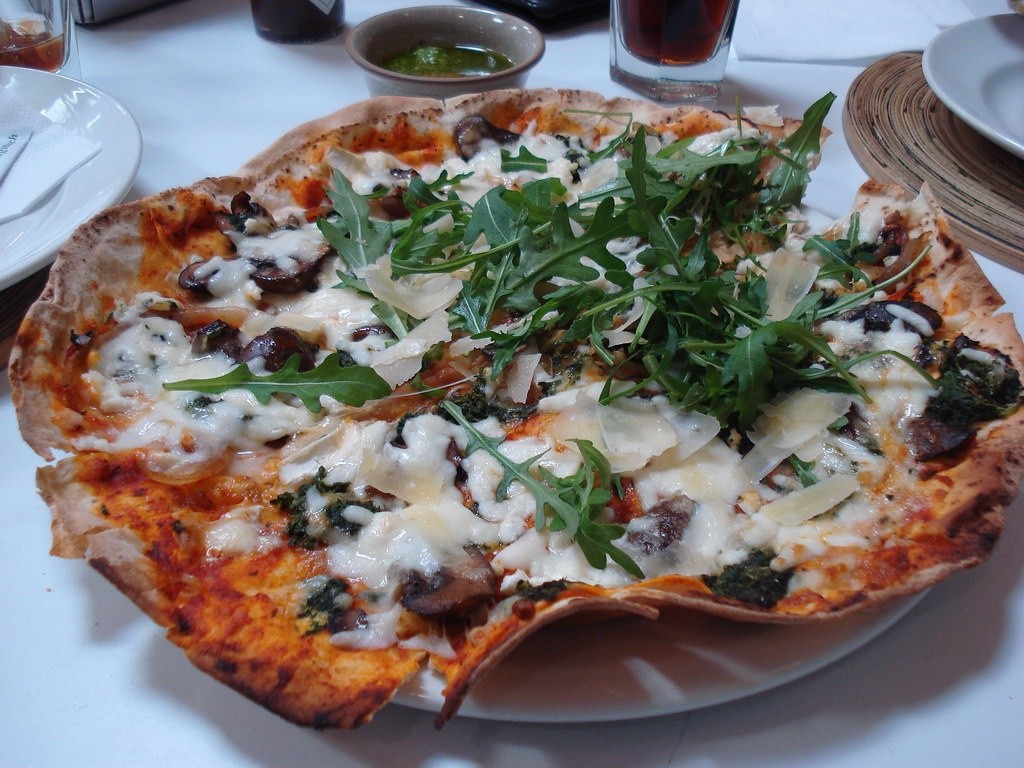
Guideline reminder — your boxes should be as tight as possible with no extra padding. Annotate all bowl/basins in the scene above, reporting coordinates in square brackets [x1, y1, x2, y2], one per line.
[345, 5, 546, 101]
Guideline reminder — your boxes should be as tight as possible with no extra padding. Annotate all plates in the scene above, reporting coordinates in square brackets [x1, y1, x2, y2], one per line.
[389, 199, 932, 725]
[922, 11, 1023, 158]
[0, 64, 144, 298]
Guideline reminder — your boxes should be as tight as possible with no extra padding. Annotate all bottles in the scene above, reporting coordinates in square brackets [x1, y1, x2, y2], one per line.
[247, 0, 346, 44]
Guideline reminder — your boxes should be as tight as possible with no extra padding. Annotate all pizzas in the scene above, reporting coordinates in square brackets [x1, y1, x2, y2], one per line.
[8, 85, 1024, 727]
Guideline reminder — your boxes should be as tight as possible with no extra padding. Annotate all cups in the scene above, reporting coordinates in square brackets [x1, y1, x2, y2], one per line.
[608, 0, 740, 101]
[0, 1, 82, 84]
